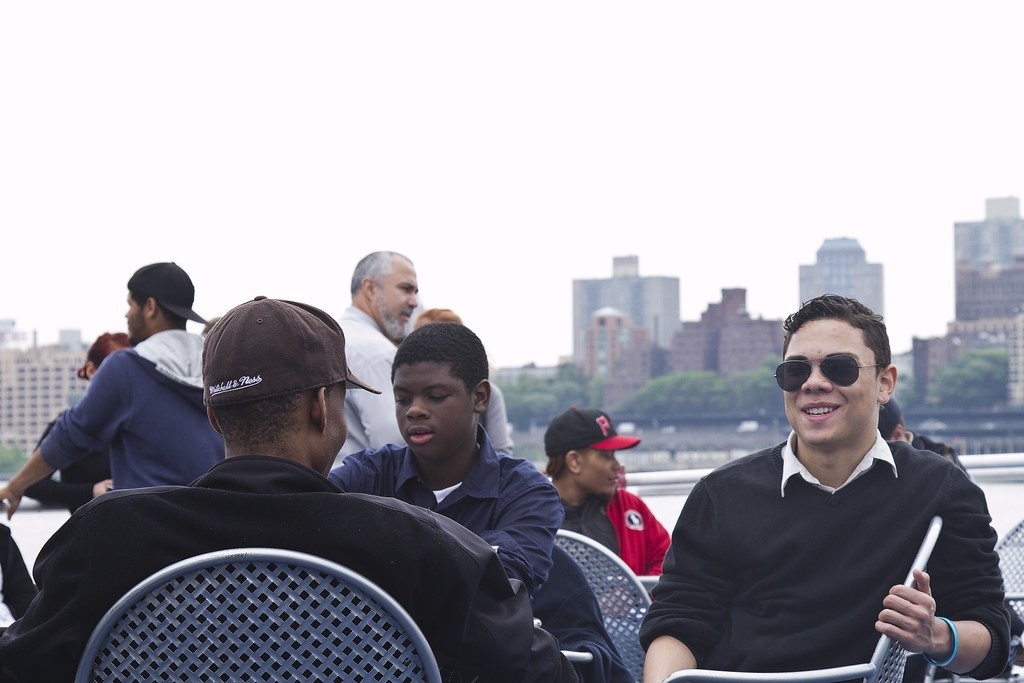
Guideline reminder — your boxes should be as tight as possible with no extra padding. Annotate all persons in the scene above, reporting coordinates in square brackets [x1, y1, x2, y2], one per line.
[327, 323, 564, 608]
[878, 397, 972, 482]
[328, 250, 514, 471]
[545, 406, 671, 623]
[638, 295, 1011, 683]
[0, 262, 226, 627]
[0, 296, 580, 683]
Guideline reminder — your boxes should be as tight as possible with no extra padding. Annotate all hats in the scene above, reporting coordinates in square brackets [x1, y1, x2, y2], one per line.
[131, 259, 204, 324]
[546, 407, 643, 451]
[878, 397, 902, 436]
[204, 295, 381, 398]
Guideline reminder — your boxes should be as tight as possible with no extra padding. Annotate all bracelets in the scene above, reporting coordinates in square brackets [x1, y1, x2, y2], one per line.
[922, 617, 959, 667]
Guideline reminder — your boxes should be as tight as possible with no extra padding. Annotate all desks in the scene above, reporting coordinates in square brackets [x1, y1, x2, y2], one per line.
[553, 529, 653, 683]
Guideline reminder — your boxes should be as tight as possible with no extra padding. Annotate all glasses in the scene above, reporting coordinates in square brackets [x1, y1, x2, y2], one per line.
[773, 352, 884, 394]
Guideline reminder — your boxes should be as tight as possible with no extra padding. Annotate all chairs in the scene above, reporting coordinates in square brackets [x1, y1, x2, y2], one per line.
[993, 519, 1024, 623]
[74, 547, 443, 683]
[661, 515, 943, 682]
